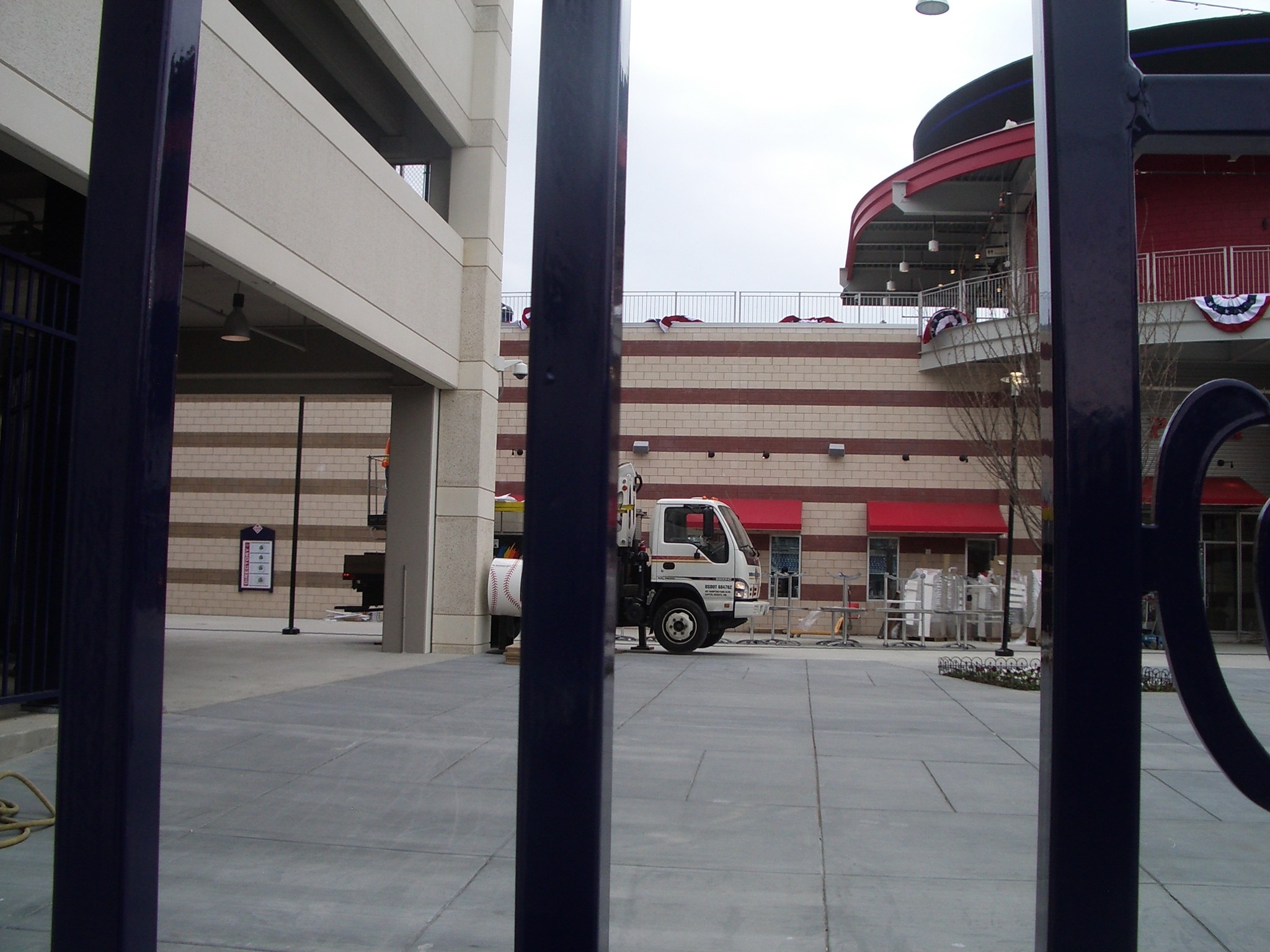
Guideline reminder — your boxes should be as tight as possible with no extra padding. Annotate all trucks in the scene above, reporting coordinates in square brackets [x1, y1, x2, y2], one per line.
[339, 454, 771, 655]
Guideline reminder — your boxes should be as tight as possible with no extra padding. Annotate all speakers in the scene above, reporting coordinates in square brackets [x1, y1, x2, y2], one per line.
[632, 441, 649, 453]
[829, 444, 845, 456]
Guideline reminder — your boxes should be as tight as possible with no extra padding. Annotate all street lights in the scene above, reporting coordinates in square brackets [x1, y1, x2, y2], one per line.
[993, 371, 1030, 657]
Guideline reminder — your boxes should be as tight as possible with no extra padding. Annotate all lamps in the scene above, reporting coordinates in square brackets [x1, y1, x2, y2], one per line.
[828, 443, 845, 457]
[221, 280, 251, 341]
[632, 440, 650, 454]
[883, 265, 896, 305]
[928, 214, 939, 251]
[899, 245, 909, 273]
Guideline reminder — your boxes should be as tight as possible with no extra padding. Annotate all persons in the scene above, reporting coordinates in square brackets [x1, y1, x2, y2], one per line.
[781, 567, 788, 597]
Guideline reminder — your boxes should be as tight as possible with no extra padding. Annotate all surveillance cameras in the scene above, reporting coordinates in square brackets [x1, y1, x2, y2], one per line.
[512, 364, 528, 380]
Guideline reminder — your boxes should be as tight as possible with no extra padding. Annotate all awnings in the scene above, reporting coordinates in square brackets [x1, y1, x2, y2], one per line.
[687, 497, 802, 531]
[867, 501, 1008, 534]
[1142, 476, 1268, 505]
